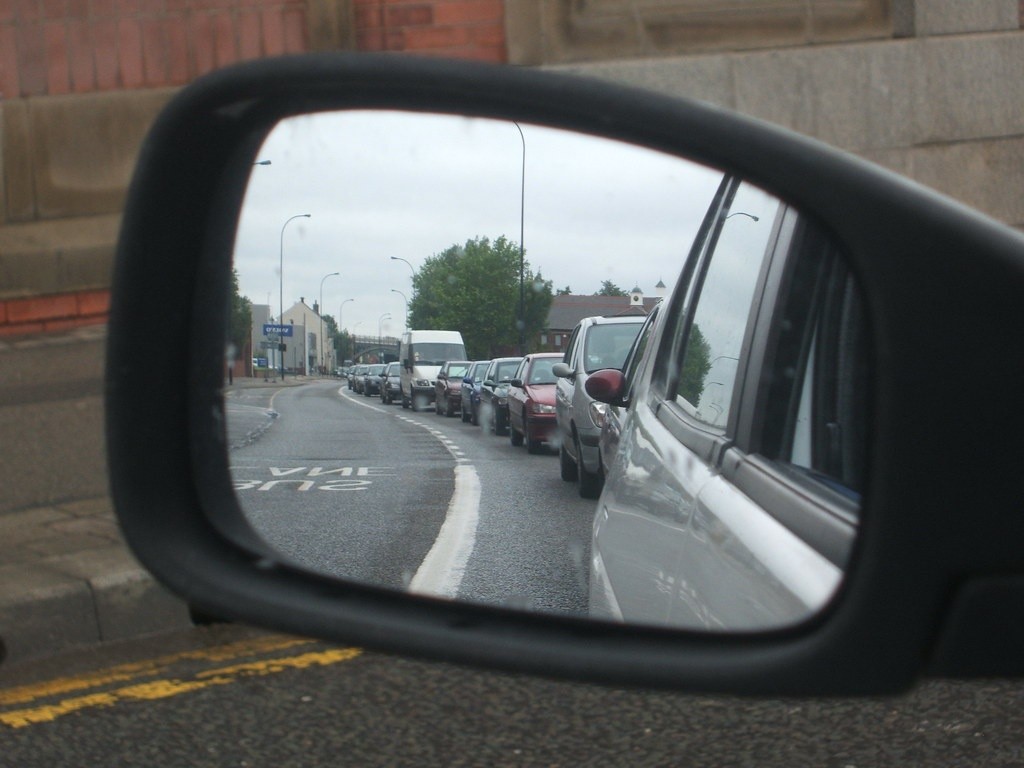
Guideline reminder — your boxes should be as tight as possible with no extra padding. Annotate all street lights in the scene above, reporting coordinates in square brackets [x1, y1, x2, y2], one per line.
[391, 257, 416, 328]
[377, 312, 392, 365]
[280, 214, 310, 381]
[321, 272, 355, 375]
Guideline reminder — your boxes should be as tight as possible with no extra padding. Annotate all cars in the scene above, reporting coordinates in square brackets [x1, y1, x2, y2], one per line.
[436, 353, 599, 455]
[347, 362, 400, 404]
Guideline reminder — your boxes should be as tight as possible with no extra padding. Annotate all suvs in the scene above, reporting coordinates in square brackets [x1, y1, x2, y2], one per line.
[552, 314, 655, 498]
[588, 150, 859, 652]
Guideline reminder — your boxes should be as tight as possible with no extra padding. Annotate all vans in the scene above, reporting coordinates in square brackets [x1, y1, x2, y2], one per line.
[400, 330, 468, 412]
